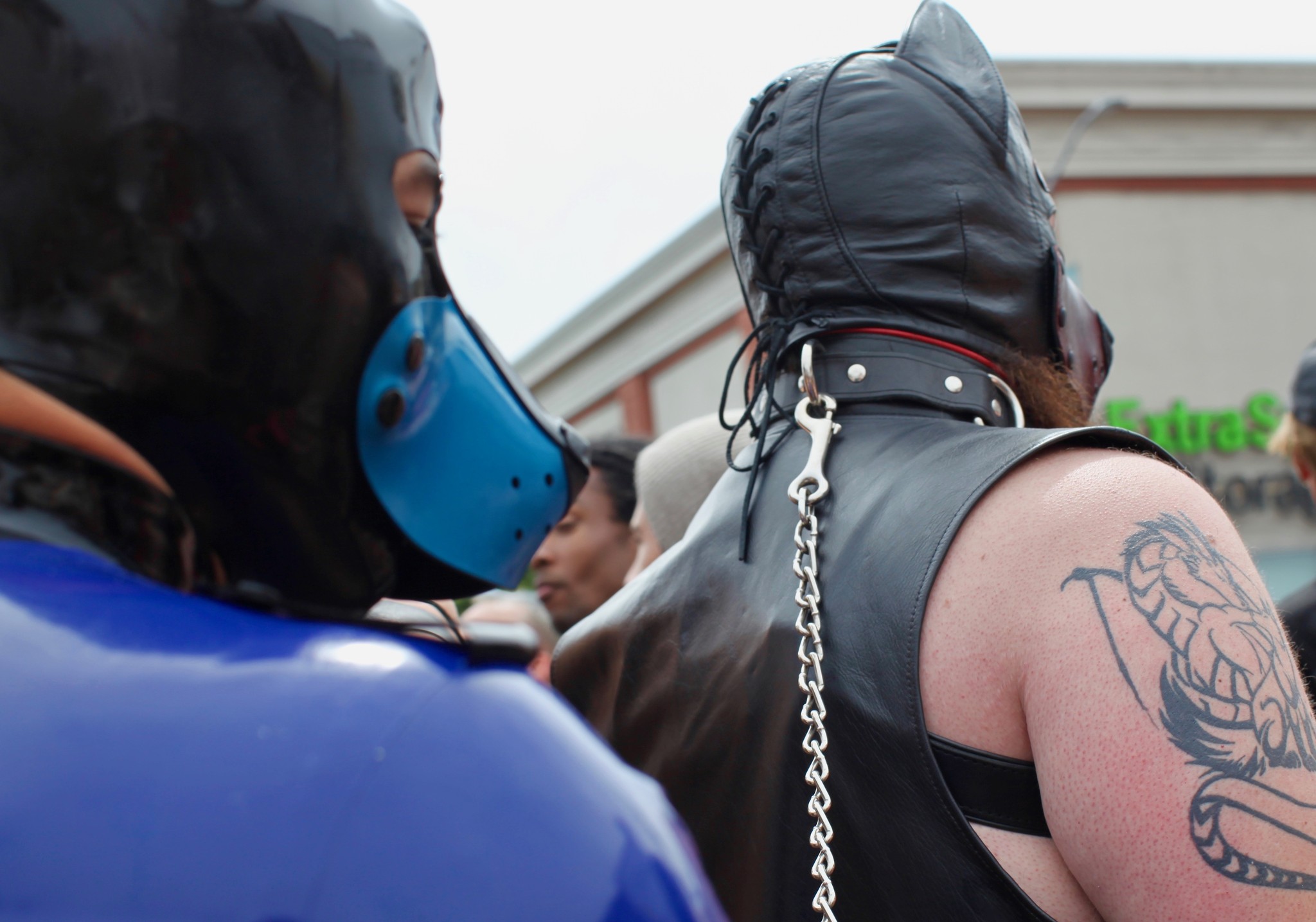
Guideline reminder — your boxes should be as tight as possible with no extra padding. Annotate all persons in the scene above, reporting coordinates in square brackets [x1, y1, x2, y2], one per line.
[457, 585, 556, 687]
[1, 1, 726, 922]
[385, 595, 457, 642]
[533, 435, 654, 632]
[622, 404, 761, 592]
[556, 1, 1315, 922]
[1274, 359, 1316, 713]
